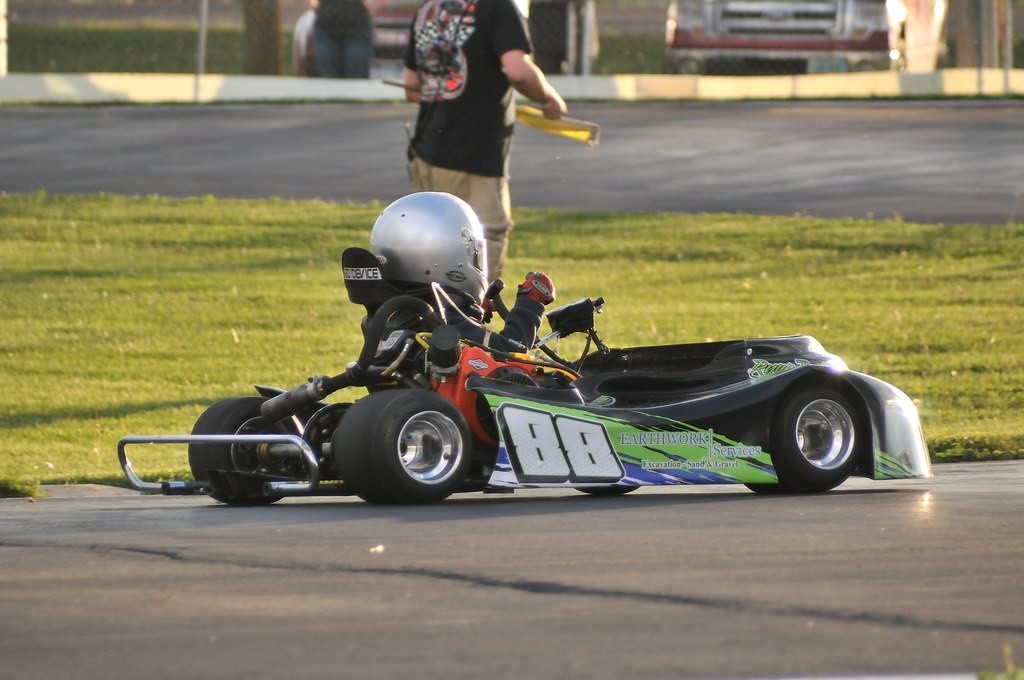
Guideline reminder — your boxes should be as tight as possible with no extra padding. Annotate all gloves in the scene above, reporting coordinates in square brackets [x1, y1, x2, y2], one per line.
[519, 272, 556, 305]
[483, 299, 495, 323]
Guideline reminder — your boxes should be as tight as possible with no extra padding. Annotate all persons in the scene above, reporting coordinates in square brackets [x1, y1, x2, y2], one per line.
[293, 0, 372, 78]
[403, 0, 568, 285]
[371, 191, 555, 361]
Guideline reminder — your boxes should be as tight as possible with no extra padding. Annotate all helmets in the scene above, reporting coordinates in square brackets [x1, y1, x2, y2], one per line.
[371, 191, 489, 306]
